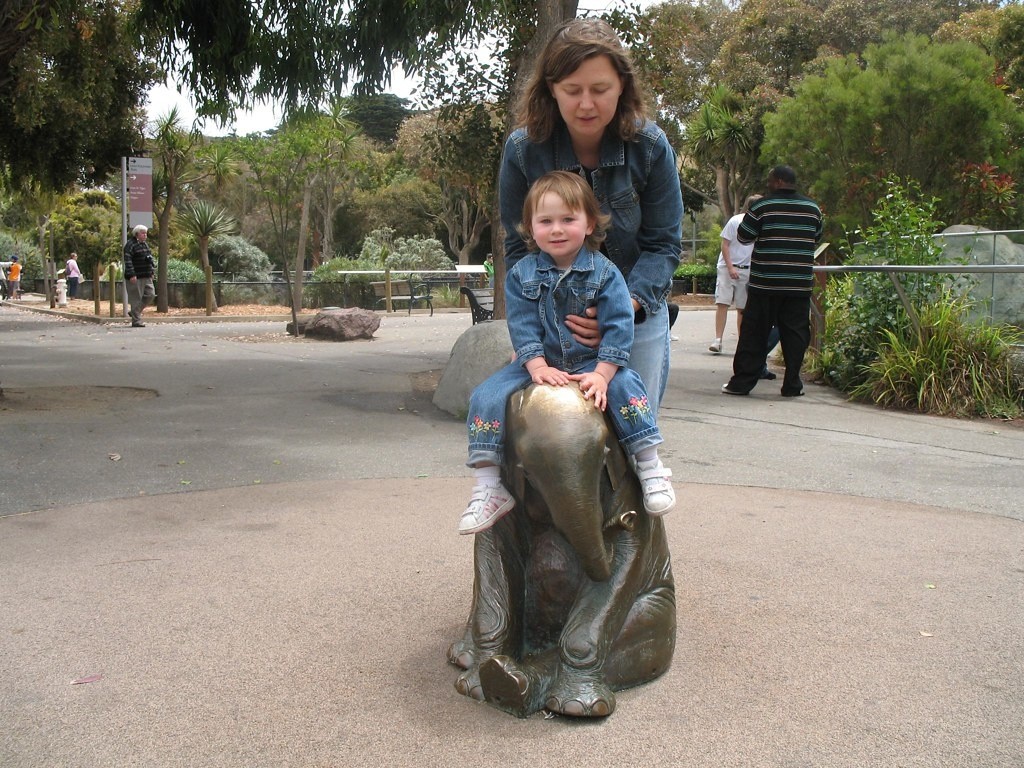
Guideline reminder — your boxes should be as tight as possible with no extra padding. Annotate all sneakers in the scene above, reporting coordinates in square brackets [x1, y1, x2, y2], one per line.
[634, 461, 676, 516]
[458, 483, 516, 535]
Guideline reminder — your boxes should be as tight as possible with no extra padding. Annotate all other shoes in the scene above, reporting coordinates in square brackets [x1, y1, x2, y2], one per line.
[721, 383, 750, 395]
[761, 371, 777, 379]
[670, 333, 679, 341]
[128, 311, 133, 318]
[708, 343, 723, 353]
[71, 297, 76, 300]
[132, 322, 146, 327]
[781, 385, 806, 396]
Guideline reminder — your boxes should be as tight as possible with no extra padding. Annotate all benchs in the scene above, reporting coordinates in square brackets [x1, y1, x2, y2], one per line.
[460, 285, 494, 325]
[368, 278, 433, 317]
[0, 278, 24, 300]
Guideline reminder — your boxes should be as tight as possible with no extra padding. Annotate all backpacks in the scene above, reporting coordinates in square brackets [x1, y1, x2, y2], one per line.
[65, 261, 76, 275]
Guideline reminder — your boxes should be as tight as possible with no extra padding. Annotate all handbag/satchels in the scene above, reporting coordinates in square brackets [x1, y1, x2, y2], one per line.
[78, 274, 85, 284]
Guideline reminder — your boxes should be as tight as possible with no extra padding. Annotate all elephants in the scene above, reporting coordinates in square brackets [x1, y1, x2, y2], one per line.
[444, 378, 677, 718]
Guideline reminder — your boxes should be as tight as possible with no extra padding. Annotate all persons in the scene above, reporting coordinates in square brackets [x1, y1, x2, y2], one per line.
[457, 170, 676, 536]
[497, 16, 682, 424]
[64, 253, 82, 300]
[0, 255, 23, 301]
[667, 164, 823, 395]
[122, 224, 155, 327]
